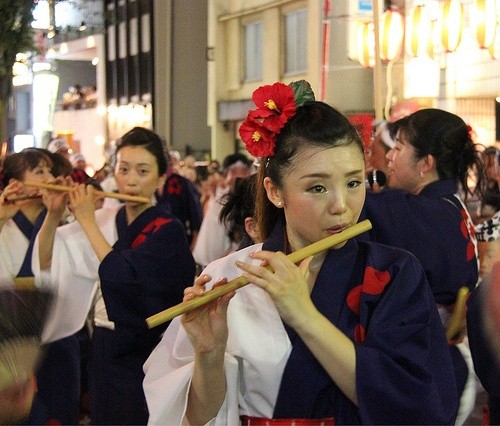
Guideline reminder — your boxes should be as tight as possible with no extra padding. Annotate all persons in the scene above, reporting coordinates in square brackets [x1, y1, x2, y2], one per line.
[31, 126, 195, 426]
[23, 139, 120, 226]
[153, 149, 225, 252]
[218, 174, 258, 253]
[143, 79, 460, 425]
[192, 152, 257, 274]
[369, 122, 397, 178]
[475, 145, 500, 233]
[477, 239, 500, 281]
[352, 109, 480, 426]
[0, 148, 97, 425]
[62, 84, 97, 112]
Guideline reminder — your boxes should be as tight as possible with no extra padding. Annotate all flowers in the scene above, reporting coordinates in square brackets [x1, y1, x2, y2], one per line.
[238, 79, 315, 158]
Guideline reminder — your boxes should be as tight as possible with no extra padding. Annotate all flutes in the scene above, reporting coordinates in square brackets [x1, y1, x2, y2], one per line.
[24, 180, 149, 204]
[5, 193, 41, 202]
[145, 219, 373, 328]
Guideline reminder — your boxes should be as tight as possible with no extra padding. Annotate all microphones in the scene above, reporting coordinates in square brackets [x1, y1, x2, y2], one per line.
[365, 169, 386, 189]
